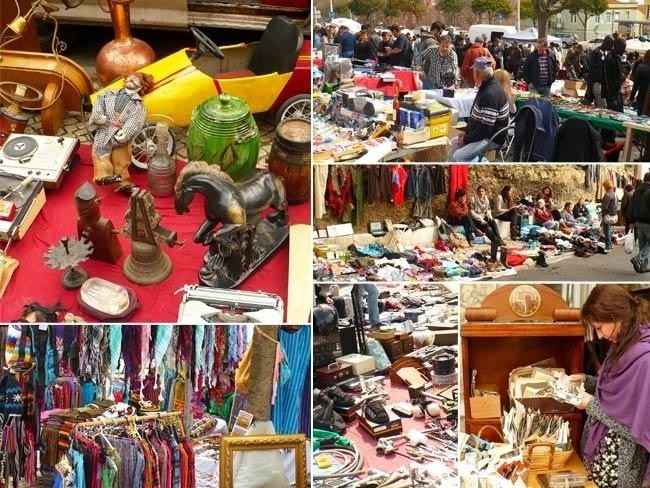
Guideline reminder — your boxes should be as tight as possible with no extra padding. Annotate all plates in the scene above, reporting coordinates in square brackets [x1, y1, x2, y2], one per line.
[429, 371, 458, 387]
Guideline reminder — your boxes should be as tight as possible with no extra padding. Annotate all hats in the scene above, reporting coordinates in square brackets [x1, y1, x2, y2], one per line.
[469, 57, 491, 69]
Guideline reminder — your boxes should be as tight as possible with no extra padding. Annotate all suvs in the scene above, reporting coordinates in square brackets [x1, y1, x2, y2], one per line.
[549, 31, 578, 49]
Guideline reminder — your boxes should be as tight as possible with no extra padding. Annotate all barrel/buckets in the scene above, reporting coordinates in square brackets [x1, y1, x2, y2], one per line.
[268, 117, 310, 205]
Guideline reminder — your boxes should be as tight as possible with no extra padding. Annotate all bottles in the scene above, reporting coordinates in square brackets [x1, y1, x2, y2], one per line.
[146, 119, 179, 197]
[393, 93, 401, 123]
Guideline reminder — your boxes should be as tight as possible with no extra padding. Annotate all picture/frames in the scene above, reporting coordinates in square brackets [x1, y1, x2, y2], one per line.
[368, 220, 383, 233]
[219, 434, 307, 488]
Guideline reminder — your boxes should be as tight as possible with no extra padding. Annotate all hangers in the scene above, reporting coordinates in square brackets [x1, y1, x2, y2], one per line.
[84, 413, 185, 473]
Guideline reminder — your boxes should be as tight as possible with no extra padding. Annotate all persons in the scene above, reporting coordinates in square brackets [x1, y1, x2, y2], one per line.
[447, 173, 650, 273]
[314, 22, 650, 162]
[570, 284, 650, 488]
[87, 72, 154, 193]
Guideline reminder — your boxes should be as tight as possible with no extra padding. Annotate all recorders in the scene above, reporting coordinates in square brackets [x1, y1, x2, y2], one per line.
[348, 89, 383, 115]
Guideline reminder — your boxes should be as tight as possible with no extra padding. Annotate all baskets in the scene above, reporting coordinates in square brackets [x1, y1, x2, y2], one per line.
[522, 441, 574, 471]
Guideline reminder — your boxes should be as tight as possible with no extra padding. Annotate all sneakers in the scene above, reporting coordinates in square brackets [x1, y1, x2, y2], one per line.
[365, 401, 390, 424]
[474, 251, 505, 273]
[475, 230, 486, 237]
[327, 387, 355, 405]
[631, 255, 641, 273]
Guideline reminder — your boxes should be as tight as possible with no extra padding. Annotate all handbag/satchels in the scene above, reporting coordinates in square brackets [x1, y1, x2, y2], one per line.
[604, 215, 618, 225]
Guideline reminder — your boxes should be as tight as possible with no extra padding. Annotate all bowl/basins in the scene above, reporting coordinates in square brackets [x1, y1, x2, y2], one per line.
[431, 353, 456, 374]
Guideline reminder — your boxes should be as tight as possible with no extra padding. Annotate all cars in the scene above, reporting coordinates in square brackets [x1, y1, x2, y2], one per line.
[575, 36, 605, 51]
[376, 26, 470, 38]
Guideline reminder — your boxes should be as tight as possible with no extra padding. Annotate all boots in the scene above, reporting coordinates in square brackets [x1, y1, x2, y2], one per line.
[500, 247, 512, 269]
[488, 243, 498, 263]
[536, 251, 548, 267]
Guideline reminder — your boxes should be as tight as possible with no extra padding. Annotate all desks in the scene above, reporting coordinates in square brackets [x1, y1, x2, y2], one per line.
[517, 91, 628, 132]
[422, 88, 478, 117]
[313, 105, 451, 162]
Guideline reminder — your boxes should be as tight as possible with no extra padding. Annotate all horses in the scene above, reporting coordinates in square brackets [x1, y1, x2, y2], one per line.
[173, 160, 289, 258]
